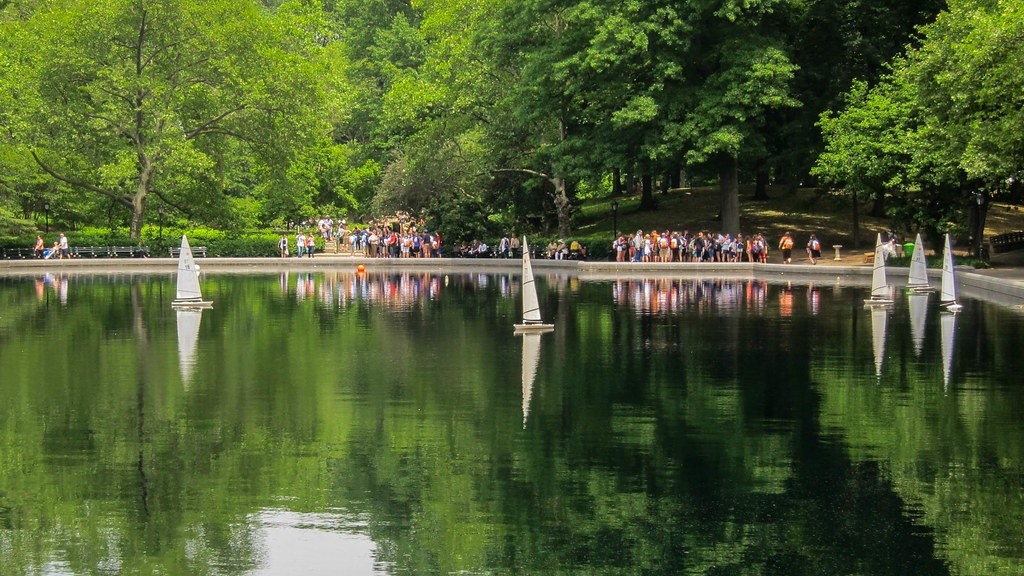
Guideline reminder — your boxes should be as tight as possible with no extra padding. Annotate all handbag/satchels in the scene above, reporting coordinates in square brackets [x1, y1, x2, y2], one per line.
[508, 248, 514, 258]
[284, 248, 290, 256]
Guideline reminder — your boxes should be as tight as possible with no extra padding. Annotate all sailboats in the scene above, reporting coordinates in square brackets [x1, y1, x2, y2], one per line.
[939, 233, 963, 312]
[513, 235, 556, 330]
[905, 233, 937, 292]
[170, 235, 215, 307]
[862, 233, 896, 303]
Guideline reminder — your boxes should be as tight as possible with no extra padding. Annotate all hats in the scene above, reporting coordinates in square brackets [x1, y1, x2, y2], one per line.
[557, 238, 565, 245]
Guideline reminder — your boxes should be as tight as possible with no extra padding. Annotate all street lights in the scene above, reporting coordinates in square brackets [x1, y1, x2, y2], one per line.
[43, 201, 51, 234]
[610, 199, 619, 240]
[158, 204, 165, 242]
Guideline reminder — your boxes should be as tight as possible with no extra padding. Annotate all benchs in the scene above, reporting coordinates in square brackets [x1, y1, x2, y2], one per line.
[452, 245, 588, 260]
[74, 246, 113, 258]
[111, 246, 150, 258]
[168, 246, 207, 258]
[0, 247, 75, 259]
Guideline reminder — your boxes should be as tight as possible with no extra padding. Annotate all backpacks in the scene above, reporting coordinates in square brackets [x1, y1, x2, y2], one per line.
[612, 239, 619, 249]
[403, 237, 410, 248]
[783, 237, 794, 250]
[811, 240, 820, 251]
[660, 238, 668, 248]
[363, 234, 370, 245]
[503, 237, 509, 248]
[670, 237, 678, 249]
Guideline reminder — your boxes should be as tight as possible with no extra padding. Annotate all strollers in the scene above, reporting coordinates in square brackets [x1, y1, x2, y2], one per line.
[40, 242, 63, 259]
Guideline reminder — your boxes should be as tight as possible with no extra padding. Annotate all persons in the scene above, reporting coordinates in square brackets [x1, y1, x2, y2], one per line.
[886, 239, 895, 248]
[278, 215, 823, 263]
[278, 272, 519, 312]
[547, 273, 579, 297]
[135, 242, 147, 259]
[34, 233, 70, 259]
[36, 274, 68, 309]
[612, 278, 820, 318]
